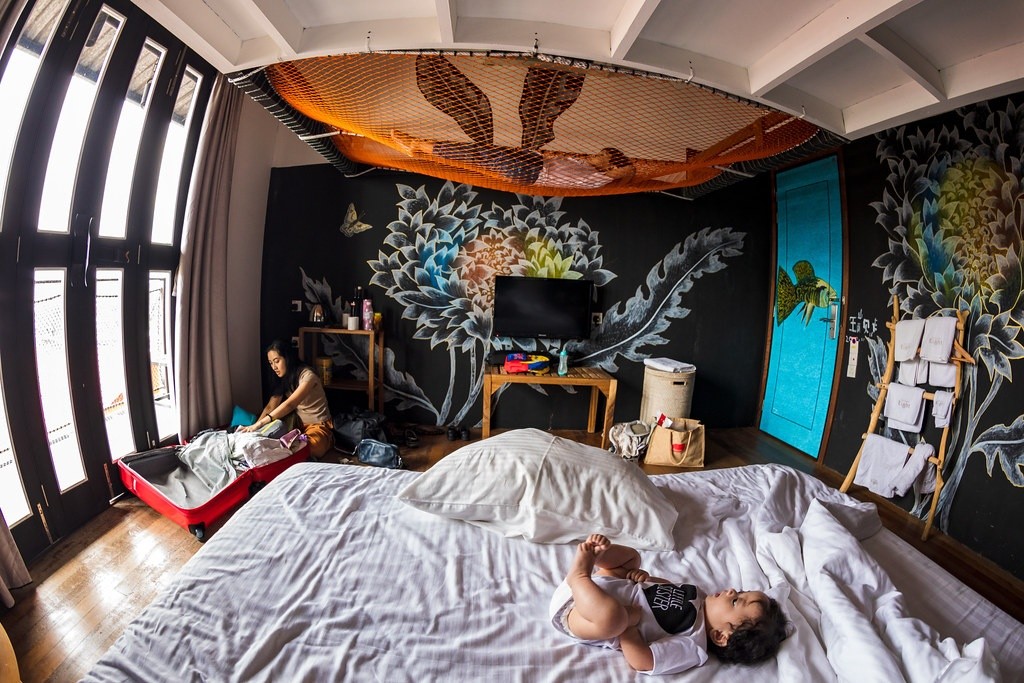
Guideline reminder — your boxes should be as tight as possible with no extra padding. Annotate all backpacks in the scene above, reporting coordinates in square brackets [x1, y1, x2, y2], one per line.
[504, 353, 550, 374]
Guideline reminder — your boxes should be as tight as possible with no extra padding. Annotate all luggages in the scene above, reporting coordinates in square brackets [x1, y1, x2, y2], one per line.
[118, 424, 312, 540]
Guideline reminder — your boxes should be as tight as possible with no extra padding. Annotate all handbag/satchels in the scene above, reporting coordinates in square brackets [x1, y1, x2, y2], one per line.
[642, 416, 704, 468]
[354, 439, 403, 468]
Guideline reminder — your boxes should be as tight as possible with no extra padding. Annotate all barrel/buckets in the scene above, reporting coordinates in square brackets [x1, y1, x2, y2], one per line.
[640, 366, 695, 426]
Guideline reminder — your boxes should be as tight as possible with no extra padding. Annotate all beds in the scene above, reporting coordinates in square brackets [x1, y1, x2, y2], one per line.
[87, 454, 1024, 683]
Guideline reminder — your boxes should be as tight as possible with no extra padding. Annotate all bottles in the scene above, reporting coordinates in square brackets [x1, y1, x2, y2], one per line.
[558, 348, 568, 376]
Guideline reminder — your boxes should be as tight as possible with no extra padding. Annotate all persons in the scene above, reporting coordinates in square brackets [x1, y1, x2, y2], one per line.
[391, 128, 637, 192]
[547, 530, 787, 676]
[235, 340, 335, 460]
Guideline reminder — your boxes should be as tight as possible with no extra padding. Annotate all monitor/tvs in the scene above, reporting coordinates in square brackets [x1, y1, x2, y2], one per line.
[493, 275, 594, 340]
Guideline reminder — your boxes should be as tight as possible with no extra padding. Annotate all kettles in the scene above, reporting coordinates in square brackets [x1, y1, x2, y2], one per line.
[308, 303, 329, 328]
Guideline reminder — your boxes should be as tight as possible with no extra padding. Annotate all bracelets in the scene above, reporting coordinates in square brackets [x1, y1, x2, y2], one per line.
[266, 413, 273, 422]
